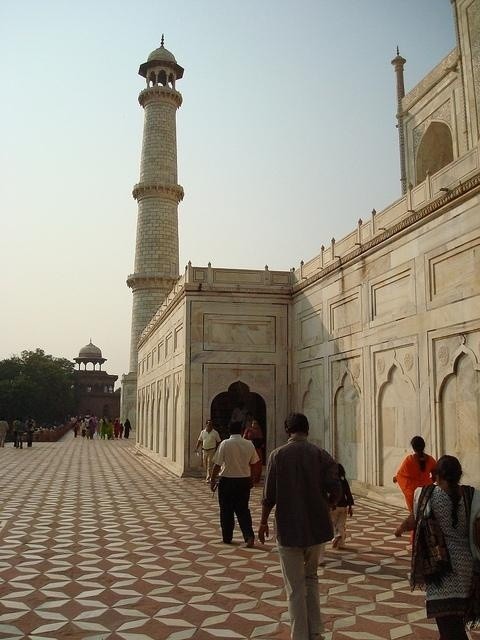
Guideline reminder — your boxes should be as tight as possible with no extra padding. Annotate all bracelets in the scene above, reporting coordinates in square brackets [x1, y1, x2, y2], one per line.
[260, 524, 268, 528]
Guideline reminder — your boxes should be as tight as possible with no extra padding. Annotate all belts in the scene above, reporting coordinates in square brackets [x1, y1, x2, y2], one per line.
[202, 448, 214, 450]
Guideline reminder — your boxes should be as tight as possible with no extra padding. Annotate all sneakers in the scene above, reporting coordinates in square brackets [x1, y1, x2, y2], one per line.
[332, 535, 340, 548]
[246, 535, 254, 547]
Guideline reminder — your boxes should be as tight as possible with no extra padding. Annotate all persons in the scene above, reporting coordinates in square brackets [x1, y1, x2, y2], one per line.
[394, 454, 480, 640]
[331, 462, 354, 550]
[258, 412, 342, 640]
[242, 415, 265, 483]
[195, 420, 221, 483]
[210, 421, 262, 549]
[1, 413, 133, 449]
[393, 436, 438, 545]
